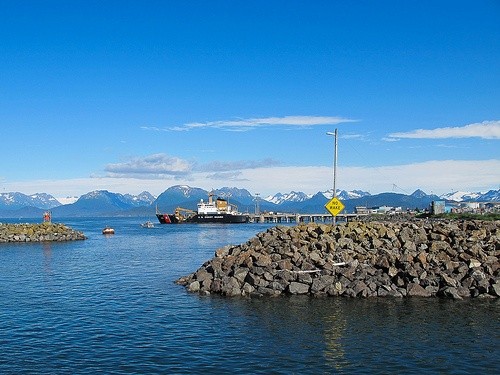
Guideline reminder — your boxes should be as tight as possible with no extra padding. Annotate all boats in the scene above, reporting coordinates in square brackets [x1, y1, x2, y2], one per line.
[156, 193, 249, 223]
